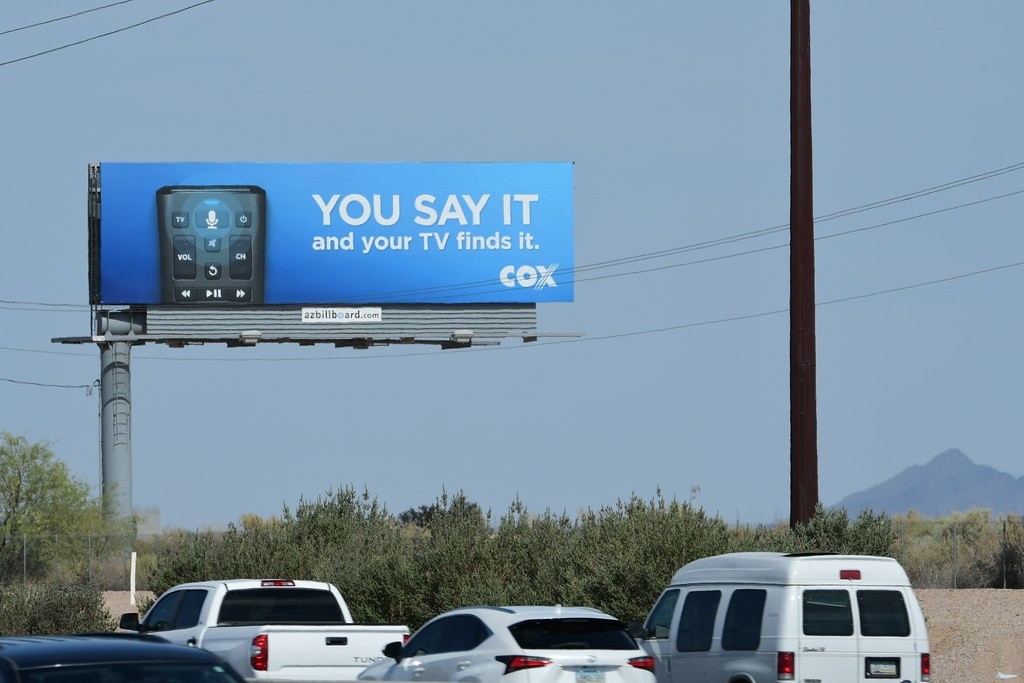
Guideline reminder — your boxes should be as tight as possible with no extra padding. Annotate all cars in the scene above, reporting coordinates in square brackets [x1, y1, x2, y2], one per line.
[357, 603, 657, 683]
[0, 632, 248, 683]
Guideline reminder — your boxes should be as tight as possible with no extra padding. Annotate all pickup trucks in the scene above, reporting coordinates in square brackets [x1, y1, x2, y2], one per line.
[118, 578, 411, 683]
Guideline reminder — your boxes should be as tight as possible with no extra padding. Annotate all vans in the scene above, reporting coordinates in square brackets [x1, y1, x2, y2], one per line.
[626, 551, 932, 683]
